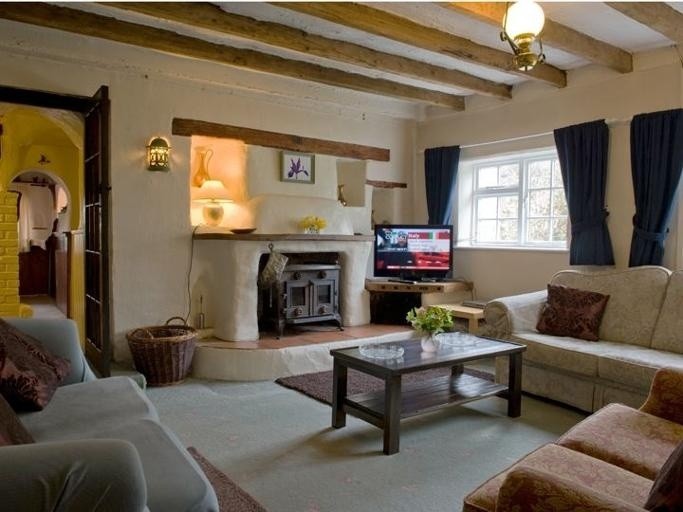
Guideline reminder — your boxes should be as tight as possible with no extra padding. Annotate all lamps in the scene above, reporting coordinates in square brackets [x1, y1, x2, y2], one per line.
[191, 180, 235, 226]
[498, 1, 546, 73]
[145, 138, 168, 169]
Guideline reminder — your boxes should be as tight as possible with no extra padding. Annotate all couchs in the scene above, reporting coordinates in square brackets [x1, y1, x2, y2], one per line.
[459, 364, 682, 512]
[2, 317, 221, 512]
[477, 264, 682, 414]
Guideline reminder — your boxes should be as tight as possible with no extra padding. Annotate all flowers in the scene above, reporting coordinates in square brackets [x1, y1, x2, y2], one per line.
[297, 216, 326, 227]
[405, 305, 452, 346]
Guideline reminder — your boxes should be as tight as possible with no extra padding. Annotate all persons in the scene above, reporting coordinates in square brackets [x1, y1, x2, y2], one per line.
[391, 231, 408, 247]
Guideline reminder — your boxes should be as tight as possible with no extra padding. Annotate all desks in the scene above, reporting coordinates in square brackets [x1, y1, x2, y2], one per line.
[430, 300, 486, 336]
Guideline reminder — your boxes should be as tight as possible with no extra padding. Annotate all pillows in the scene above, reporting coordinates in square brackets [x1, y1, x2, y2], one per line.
[2, 393, 37, 445]
[534, 282, 610, 344]
[0, 320, 71, 410]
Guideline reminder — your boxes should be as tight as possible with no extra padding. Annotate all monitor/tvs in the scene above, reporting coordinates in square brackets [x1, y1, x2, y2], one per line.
[374, 224, 453, 283]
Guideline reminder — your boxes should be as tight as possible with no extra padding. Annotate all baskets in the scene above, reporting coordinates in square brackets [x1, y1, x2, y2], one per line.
[125, 316, 197, 386]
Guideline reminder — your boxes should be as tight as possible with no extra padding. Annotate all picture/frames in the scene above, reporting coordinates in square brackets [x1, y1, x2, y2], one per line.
[280, 151, 317, 185]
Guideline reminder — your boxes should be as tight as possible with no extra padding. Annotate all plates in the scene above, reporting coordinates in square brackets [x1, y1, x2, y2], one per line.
[436, 333, 481, 346]
[230, 228, 257, 234]
[358, 342, 405, 359]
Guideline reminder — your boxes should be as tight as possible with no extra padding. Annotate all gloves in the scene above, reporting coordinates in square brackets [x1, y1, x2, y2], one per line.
[256, 251, 288, 290]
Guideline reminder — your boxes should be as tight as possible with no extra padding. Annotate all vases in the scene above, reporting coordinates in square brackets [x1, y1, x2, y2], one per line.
[305, 228, 319, 235]
[419, 331, 440, 352]
[337, 184, 350, 209]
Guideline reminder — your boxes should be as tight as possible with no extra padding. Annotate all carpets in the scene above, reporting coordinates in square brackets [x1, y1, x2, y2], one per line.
[184, 446, 270, 512]
[274, 369, 495, 430]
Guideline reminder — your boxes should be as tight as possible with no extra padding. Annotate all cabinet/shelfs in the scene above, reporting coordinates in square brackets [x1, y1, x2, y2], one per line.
[16, 184, 57, 299]
[365, 277, 474, 328]
